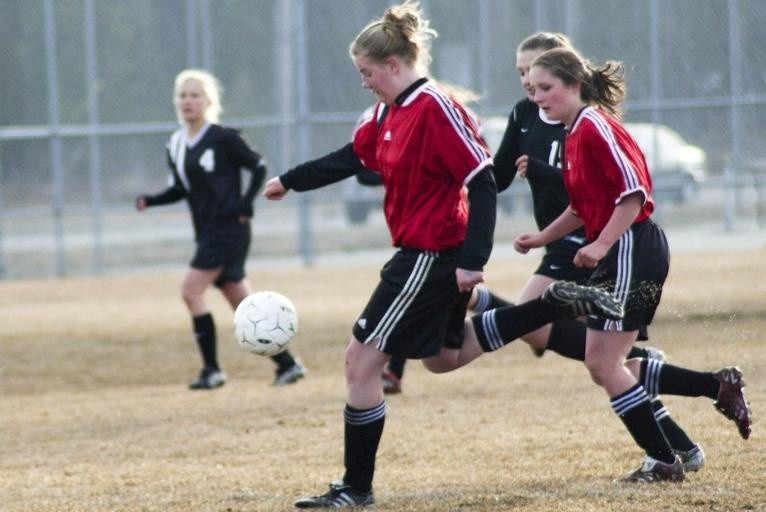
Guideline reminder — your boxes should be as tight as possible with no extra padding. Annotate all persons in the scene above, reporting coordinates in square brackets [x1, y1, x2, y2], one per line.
[514, 48, 704, 482]
[494, 31, 753, 443]
[133, 70, 308, 390]
[358, 84, 546, 394]
[263, 5, 624, 510]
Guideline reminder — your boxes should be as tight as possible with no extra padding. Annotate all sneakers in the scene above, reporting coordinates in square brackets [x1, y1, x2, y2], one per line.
[612, 442, 703, 486]
[188, 366, 227, 388]
[713, 366, 753, 440]
[272, 361, 306, 385]
[541, 280, 622, 322]
[295, 480, 375, 508]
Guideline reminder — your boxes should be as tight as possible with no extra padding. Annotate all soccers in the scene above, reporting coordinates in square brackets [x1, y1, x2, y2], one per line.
[233, 291, 298, 356]
